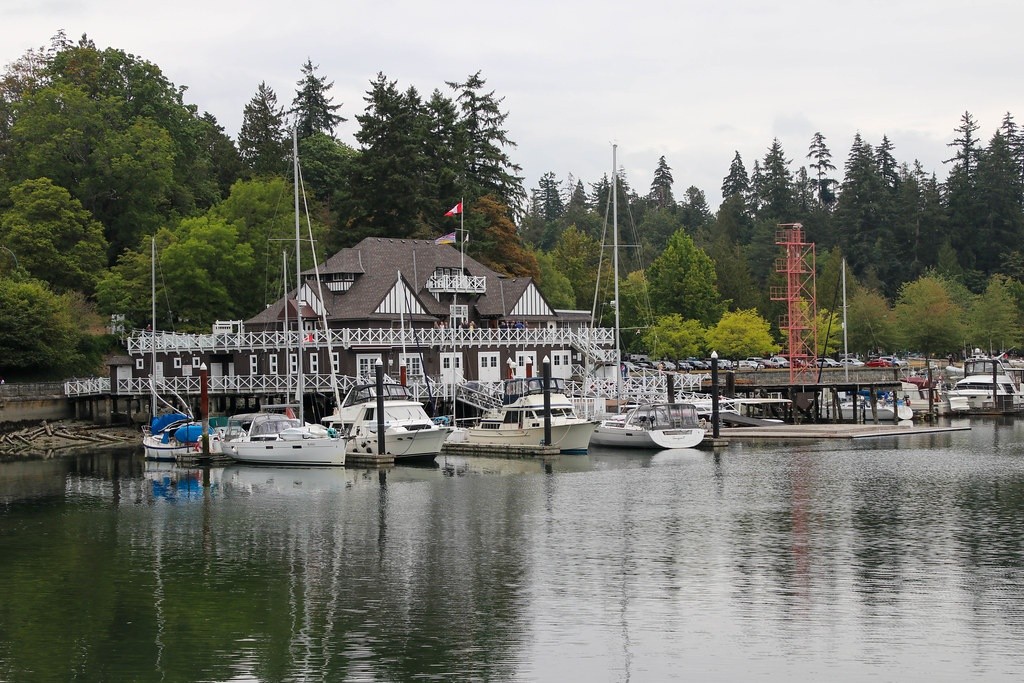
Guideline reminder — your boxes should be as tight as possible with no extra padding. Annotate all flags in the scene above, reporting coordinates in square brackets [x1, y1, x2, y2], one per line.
[304, 332, 313, 342]
[445, 202, 463, 216]
[435, 231, 458, 245]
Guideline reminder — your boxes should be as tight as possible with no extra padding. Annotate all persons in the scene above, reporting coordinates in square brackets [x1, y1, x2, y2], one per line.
[501, 320, 529, 340]
[1, 379, 5, 384]
[147, 323, 151, 331]
[434, 320, 478, 340]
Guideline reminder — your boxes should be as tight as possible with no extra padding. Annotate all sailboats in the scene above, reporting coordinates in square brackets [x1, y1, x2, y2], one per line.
[140, 127, 350, 465]
[578, 144, 708, 448]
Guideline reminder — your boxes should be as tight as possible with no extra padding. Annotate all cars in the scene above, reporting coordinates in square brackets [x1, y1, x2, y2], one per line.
[620, 354, 908, 372]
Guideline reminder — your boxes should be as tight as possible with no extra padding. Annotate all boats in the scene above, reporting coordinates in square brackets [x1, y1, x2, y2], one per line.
[441, 377, 601, 455]
[950, 346, 1024, 413]
[818, 400, 914, 420]
[317, 383, 453, 459]
